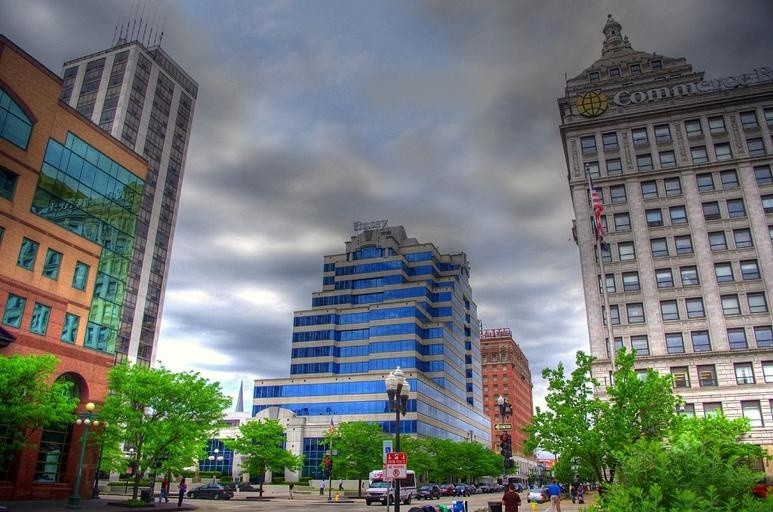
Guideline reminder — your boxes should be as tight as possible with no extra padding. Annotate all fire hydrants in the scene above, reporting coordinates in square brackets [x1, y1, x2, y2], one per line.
[334, 491, 339, 500]
[530, 501, 537, 512]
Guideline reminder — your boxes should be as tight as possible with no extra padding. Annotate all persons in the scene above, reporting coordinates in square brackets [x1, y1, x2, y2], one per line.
[500, 484, 522, 512]
[338, 478, 346, 490]
[571, 484, 576, 504]
[176, 478, 188, 507]
[159, 479, 170, 504]
[289, 481, 294, 501]
[578, 483, 585, 504]
[320, 479, 326, 497]
[548, 479, 562, 512]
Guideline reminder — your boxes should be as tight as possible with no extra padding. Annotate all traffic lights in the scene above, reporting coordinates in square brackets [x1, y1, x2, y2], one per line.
[499, 433, 510, 456]
[325, 458, 330, 471]
[328, 460, 332, 469]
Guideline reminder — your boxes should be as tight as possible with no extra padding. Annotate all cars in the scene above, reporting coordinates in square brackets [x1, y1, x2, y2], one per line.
[527, 485, 567, 503]
[752, 476, 772, 501]
[439, 483, 454, 495]
[186, 484, 233, 500]
[454, 475, 540, 500]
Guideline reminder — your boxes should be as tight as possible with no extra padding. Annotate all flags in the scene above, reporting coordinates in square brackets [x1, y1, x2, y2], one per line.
[590, 169, 604, 238]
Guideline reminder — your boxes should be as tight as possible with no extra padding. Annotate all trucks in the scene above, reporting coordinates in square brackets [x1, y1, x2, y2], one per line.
[366, 469, 416, 505]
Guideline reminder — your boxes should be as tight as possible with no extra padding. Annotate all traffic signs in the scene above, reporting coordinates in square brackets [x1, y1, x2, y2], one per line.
[493, 424, 511, 430]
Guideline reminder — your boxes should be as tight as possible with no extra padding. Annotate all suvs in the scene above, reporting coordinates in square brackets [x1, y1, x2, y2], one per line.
[417, 484, 441, 500]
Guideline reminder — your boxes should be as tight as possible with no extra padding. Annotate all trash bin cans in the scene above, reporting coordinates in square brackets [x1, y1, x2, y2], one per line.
[140, 489, 152, 502]
[488, 502, 503, 512]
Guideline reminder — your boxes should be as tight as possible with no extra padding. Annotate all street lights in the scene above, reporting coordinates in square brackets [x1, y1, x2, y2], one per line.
[208, 448, 224, 484]
[670, 405, 684, 416]
[385, 365, 411, 512]
[123, 447, 135, 494]
[496, 393, 512, 491]
[64, 402, 107, 508]
